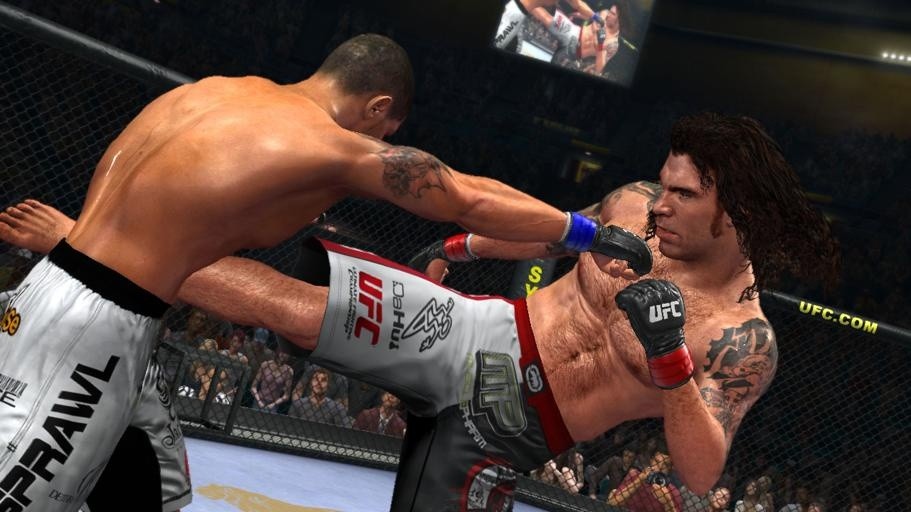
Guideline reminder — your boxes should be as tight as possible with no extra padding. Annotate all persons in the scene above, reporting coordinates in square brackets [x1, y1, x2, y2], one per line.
[1, 34, 657, 511]
[162, 304, 901, 512]
[0, 103, 782, 511]
[529, 2, 633, 77]
[492, 1, 607, 51]
